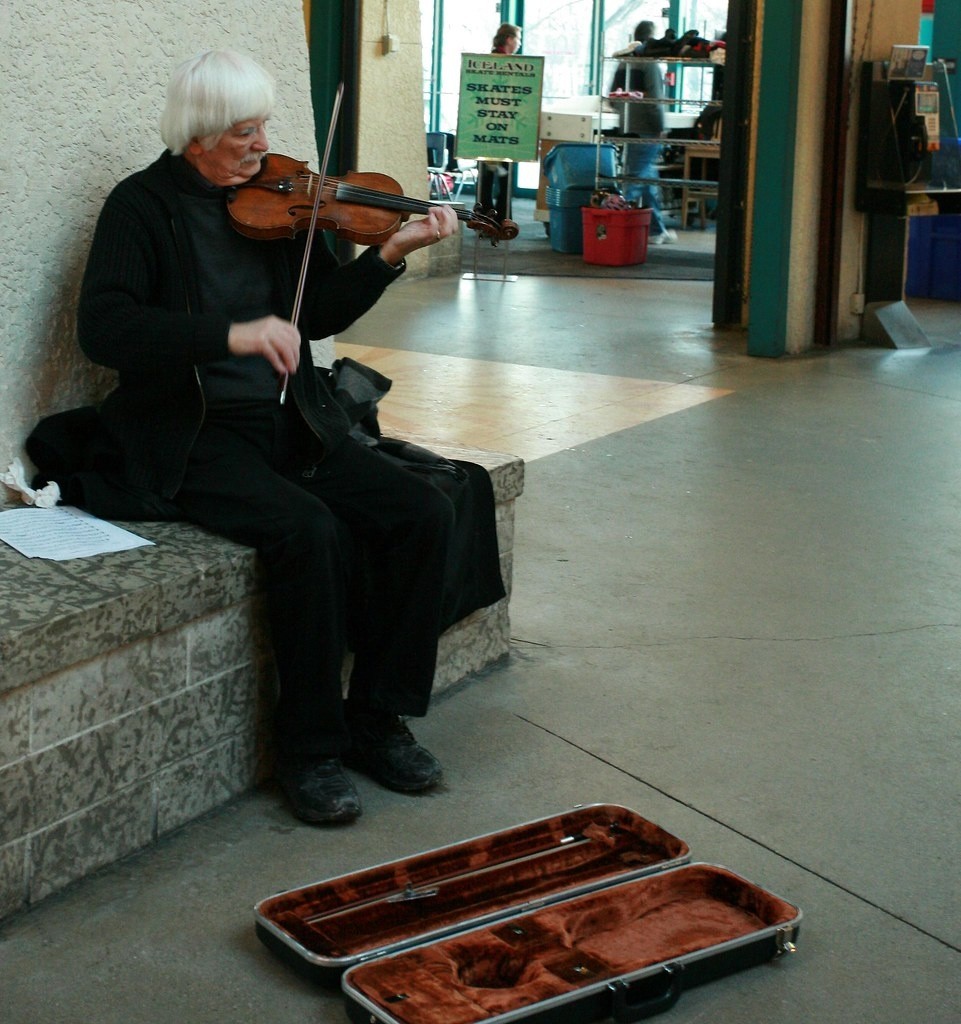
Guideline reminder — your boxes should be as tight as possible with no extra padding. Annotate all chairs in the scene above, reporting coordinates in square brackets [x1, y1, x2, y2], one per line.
[426, 131, 477, 201]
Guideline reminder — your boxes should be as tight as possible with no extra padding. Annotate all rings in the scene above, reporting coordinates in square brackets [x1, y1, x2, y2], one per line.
[436, 232, 441, 240]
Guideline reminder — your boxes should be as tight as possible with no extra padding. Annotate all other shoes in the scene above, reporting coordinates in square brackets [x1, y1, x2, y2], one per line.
[648, 230, 675, 243]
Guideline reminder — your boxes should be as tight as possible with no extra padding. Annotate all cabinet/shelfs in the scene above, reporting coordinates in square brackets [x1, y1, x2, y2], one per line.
[591, 32, 719, 193]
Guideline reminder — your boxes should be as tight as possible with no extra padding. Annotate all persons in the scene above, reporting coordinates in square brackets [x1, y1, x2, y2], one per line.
[475, 22, 522, 219]
[608, 20, 678, 245]
[25, 46, 507, 827]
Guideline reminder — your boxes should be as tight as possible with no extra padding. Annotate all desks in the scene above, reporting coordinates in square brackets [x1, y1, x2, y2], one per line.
[681, 143, 720, 229]
[532, 110, 701, 239]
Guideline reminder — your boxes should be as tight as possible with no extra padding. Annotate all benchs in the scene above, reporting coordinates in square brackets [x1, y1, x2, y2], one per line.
[0, 433, 526, 931]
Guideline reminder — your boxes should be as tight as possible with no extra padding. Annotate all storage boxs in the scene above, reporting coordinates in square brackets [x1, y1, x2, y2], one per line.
[581, 208, 653, 266]
[542, 143, 618, 253]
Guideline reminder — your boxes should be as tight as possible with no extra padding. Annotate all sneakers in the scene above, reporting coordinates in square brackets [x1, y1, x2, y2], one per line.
[270, 754, 361, 822]
[341, 710, 444, 792]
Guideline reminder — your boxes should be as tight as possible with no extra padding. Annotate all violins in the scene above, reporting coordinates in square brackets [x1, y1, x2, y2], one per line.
[223, 148, 520, 250]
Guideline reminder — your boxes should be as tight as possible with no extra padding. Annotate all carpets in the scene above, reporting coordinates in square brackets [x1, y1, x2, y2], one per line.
[453, 191, 715, 281]
